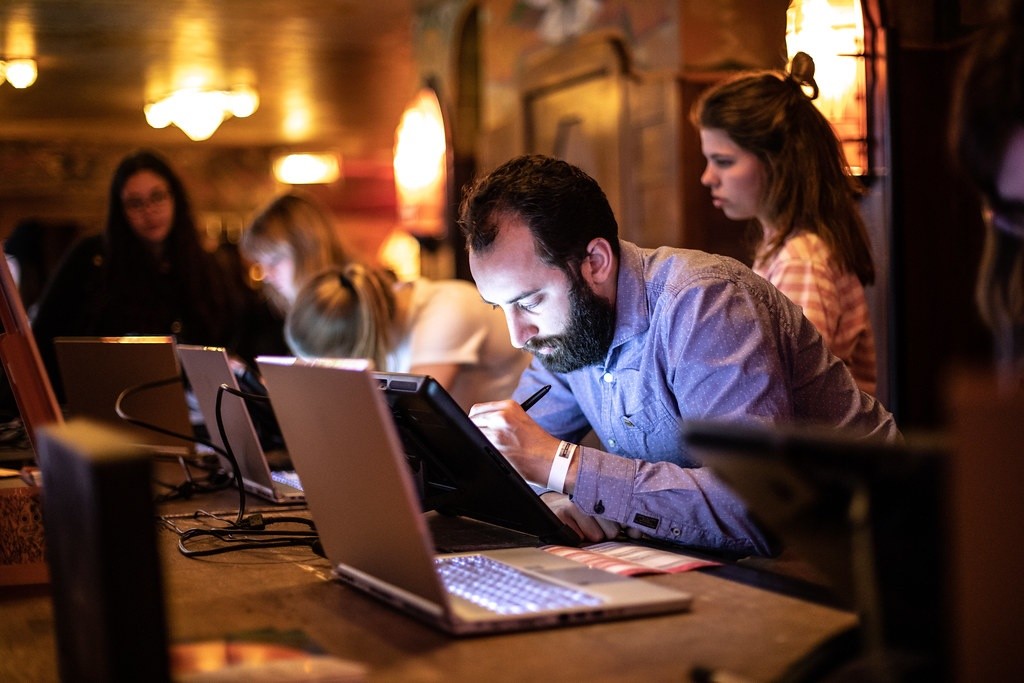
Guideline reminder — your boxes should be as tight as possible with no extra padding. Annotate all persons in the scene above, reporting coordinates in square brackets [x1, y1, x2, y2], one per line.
[690, 53, 876, 398]
[242, 194, 532, 416]
[458, 155, 907, 602]
[95, 147, 286, 358]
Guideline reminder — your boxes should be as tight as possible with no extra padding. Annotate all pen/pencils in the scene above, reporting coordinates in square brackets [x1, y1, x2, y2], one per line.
[520, 384, 552, 412]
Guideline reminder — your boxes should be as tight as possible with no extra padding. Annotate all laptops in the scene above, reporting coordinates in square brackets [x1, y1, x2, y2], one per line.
[0, 251, 693, 634]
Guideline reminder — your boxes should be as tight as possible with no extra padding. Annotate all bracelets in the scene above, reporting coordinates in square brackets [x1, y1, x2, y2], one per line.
[546, 440, 576, 493]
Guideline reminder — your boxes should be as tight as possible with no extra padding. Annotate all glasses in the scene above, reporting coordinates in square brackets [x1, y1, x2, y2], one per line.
[121, 189, 171, 215]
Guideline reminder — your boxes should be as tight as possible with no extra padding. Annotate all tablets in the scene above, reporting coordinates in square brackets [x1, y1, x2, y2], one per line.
[228, 359, 291, 454]
[371, 373, 584, 552]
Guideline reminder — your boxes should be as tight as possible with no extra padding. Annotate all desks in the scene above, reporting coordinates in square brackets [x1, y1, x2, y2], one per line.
[0, 449, 866, 683]
